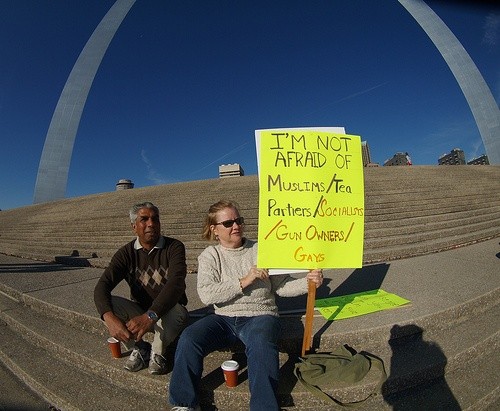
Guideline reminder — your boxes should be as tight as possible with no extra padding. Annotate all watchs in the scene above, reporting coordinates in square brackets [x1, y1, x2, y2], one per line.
[145, 311, 159, 323]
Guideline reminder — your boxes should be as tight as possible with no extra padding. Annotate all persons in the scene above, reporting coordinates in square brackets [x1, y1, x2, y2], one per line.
[93, 200, 191, 374]
[162, 200, 325, 411]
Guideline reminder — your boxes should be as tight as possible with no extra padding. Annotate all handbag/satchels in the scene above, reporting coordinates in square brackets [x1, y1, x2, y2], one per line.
[294, 345, 370, 386]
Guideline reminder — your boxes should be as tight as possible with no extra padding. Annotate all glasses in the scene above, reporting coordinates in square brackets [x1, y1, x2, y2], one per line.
[216, 217, 244, 228]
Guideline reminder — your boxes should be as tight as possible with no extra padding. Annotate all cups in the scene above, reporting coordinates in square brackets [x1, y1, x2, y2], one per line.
[221, 360, 239, 387]
[107, 336, 121, 358]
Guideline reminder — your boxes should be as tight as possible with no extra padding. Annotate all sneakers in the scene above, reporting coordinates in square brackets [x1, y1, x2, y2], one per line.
[148, 354, 166, 374]
[125, 344, 150, 372]
